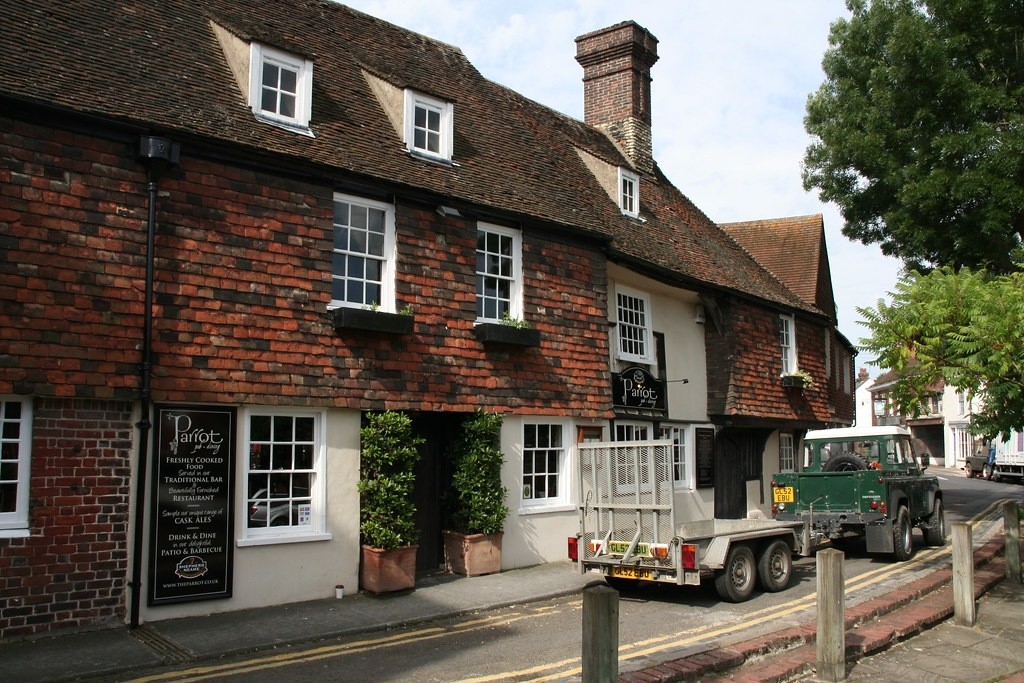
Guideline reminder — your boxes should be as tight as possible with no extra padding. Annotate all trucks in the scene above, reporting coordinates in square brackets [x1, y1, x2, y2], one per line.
[770, 425, 947, 560]
[964, 427, 1024, 483]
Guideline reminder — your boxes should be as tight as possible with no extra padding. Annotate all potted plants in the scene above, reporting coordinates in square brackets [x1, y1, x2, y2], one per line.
[334, 300, 414, 334]
[782, 370, 814, 391]
[476, 311, 541, 347]
[356, 411, 420, 594]
[440, 407, 515, 579]
